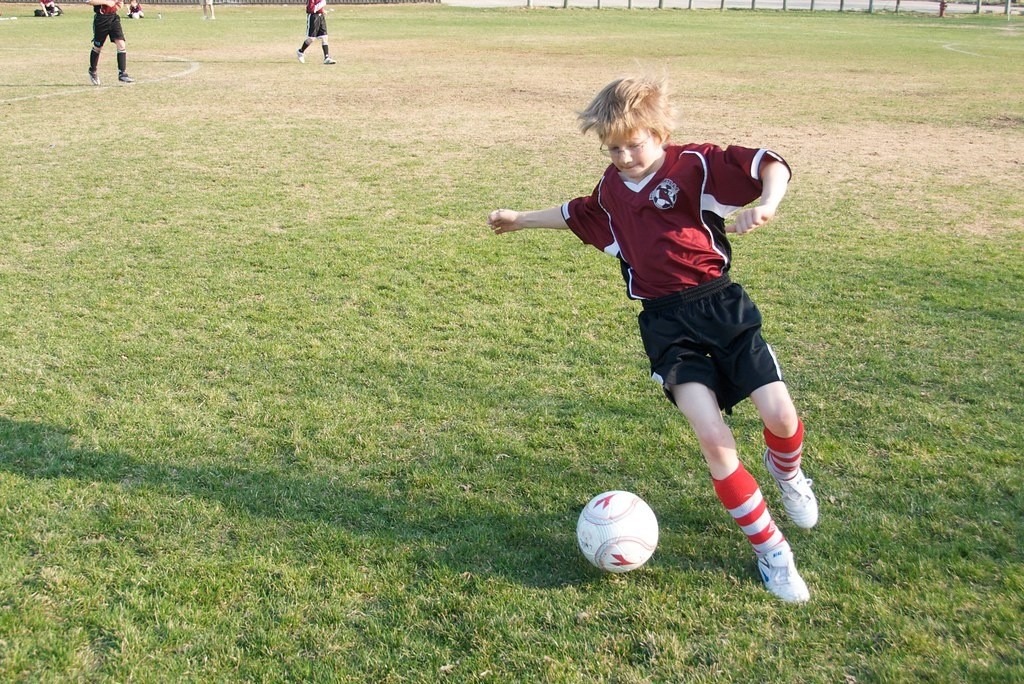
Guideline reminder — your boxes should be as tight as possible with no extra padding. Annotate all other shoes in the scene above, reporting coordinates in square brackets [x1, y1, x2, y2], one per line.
[296, 49, 306, 62]
[201, 16, 215, 20]
[118, 74, 134, 82]
[89, 69, 99, 86]
[325, 57, 338, 65]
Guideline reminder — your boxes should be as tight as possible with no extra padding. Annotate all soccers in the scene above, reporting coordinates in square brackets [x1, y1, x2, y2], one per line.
[575, 491, 659, 574]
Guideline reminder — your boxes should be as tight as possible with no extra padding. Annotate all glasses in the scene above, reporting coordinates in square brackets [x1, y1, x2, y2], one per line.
[598, 133, 656, 160]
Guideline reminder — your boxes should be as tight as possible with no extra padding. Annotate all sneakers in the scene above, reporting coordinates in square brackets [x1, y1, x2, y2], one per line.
[757, 542, 810, 605]
[761, 448, 819, 528]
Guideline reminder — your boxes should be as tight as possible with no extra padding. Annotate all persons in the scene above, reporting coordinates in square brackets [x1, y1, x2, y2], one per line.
[40, 0, 54, 18]
[296, 0, 337, 64]
[199, 0, 215, 20]
[127, 0, 144, 19]
[488, 71, 820, 605]
[85, 0, 134, 86]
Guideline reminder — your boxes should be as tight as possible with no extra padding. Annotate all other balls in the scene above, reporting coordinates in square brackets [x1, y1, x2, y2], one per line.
[132, 13, 139, 19]
